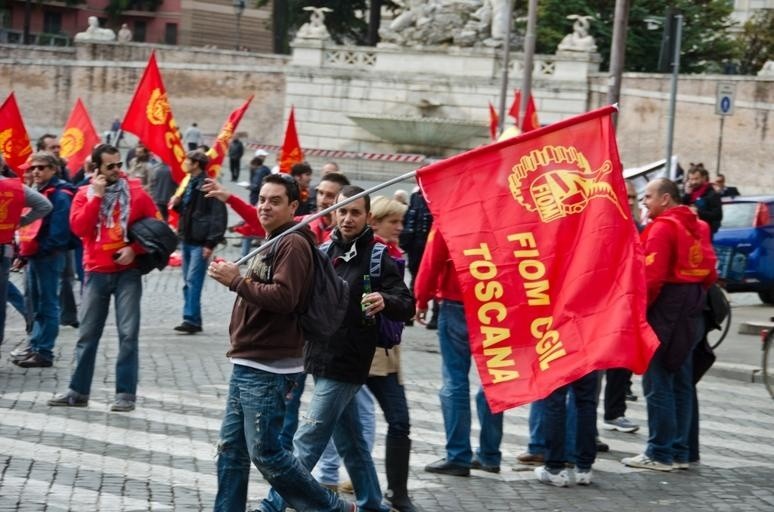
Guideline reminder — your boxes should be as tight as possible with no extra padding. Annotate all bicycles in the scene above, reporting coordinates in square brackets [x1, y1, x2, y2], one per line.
[703, 273, 730, 348]
[759, 317, 774, 394]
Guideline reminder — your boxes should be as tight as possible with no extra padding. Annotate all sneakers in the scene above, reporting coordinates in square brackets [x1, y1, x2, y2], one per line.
[11, 349, 52, 367]
[49, 390, 88, 406]
[111, 398, 135, 411]
[603, 416, 639, 432]
[622, 453, 690, 471]
[173, 321, 202, 334]
[518, 437, 608, 488]
[314, 481, 417, 512]
[425, 452, 500, 475]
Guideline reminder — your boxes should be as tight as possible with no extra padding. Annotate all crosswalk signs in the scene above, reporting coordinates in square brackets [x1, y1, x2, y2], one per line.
[716, 82, 735, 117]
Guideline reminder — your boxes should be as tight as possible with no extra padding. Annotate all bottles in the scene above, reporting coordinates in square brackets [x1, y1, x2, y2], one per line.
[360, 276, 375, 326]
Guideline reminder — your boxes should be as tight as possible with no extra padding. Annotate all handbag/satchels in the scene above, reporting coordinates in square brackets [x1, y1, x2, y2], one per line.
[704, 284, 731, 341]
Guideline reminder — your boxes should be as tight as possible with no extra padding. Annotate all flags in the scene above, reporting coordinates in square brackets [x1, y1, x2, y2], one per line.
[509, 91, 539, 131]
[417, 105, 660, 414]
[279, 111, 303, 173]
[0, 96, 34, 178]
[59, 100, 102, 175]
[120, 57, 189, 185]
[489, 107, 499, 139]
[205, 102, 247, 180]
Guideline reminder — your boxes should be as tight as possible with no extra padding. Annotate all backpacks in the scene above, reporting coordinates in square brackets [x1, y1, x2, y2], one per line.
[263, 231, 350, 338]
[369, 241, 404, 349]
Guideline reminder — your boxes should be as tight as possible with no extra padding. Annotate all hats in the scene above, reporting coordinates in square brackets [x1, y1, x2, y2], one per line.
[186, 151, 207, 162]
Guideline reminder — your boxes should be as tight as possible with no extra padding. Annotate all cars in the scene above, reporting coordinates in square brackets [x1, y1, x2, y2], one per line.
[711, 194, 774, 306]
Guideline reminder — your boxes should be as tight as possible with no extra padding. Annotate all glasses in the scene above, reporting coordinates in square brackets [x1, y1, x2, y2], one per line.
[280, 171, 298, 188]
[30, 165, 48, 170]
[106, 162, 122, 169]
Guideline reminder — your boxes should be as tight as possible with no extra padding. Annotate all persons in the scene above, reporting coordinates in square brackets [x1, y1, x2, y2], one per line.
[413, 214, 502, 475]
[0, 117, 228, 369]
[48, 143, 166, 412]
[517, 165, 738, 487]
[201, 134, 434, 511]
[119, 23, 132, 41]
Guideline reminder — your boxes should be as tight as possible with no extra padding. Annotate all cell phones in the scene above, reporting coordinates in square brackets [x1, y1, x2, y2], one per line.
[112, 252, 121, 260]
[91, 162, 100, 174]
